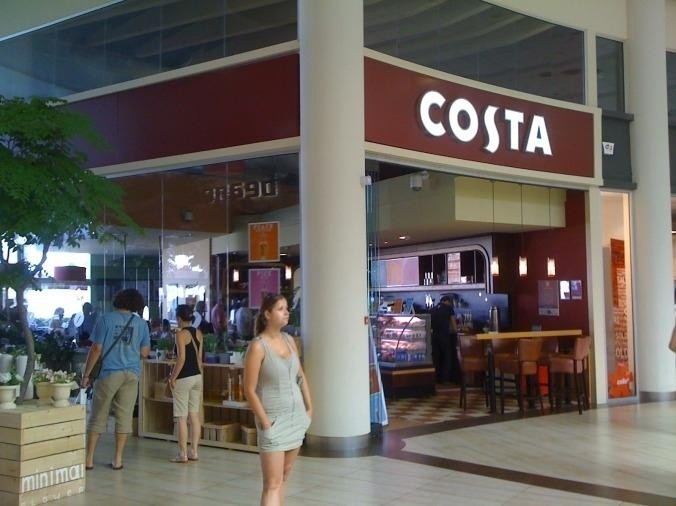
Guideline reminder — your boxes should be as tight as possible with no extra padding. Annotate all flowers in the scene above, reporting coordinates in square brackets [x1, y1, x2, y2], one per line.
[0, 371, 23, 386]
[31, 367, 56, 382]
[48, 369, 77, 384]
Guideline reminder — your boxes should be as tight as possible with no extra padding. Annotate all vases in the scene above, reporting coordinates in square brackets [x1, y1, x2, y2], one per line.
[34, 382, 52, 401]
[52, 383, 72, 407]
[0, 386, 21, 410]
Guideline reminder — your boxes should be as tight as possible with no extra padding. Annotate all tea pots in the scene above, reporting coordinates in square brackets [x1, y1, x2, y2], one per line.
[488, 305, 501, 333]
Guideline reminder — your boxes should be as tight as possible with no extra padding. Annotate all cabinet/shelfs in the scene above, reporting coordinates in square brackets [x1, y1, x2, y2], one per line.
[140, 358, 260, 455]
[370, 315, 436, 400]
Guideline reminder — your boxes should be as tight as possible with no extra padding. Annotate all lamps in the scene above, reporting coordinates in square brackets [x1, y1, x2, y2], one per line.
[490, 179, 499, 275]
[547, 186, 556, 277]
[518, 181, 527, 277]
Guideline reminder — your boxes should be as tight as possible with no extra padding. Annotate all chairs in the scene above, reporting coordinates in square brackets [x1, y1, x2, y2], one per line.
[499, 338, 544, 417]
[459, 338, 496, 413]
[549, 336, 590, 415]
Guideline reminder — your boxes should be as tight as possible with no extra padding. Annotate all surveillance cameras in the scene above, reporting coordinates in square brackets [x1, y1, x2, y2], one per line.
[182, 209, 192, 222]
[409, 174, 429, 192]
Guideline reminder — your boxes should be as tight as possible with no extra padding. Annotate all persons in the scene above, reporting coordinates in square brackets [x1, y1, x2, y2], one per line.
[78, 302, 96, 340]
[161, 319, 173, 343]
[242, 292, 312, 506]
[81, 288, 151, 472]
[196, 301, 214, 334]
[430, 296, 460, 385]
[236, 299, 254, 340]
[210, 298, 227, 338]
[168, 304, 203, 464]
[187, 298, 202, 328]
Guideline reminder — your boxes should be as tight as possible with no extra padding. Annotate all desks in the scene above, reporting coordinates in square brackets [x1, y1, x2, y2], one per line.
[476, 328, 582, 412]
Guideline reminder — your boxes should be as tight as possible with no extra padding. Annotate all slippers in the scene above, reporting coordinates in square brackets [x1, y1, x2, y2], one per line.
[169, 458, 188, 463]
[86, 467, 93, 470]
[111, 464, 123, 470]
[189, 459, 198, 461]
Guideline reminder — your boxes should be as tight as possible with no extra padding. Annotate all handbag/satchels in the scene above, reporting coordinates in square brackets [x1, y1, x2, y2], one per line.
[90, 314, 135, 378]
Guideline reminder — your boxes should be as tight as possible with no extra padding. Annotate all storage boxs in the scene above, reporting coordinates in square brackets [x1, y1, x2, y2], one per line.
[153, 381, 172, 399]
[240, 424, 257, 445]
[203, 419, 240, 443]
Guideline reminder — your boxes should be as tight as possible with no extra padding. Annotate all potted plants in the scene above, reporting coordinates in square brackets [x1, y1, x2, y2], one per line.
[217, 332, 232, 364]
[233, 345, 246, 363]
[155, 338, 176, 359]
[0, 92, 148, 506]
[0, 349, 33, 400]
[54, 265, 86, 282]
[203, 333, 218, 364]
